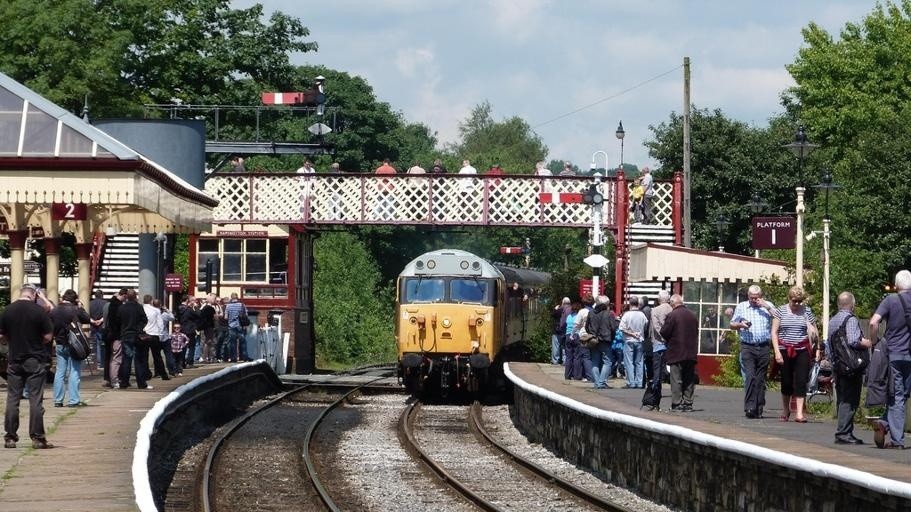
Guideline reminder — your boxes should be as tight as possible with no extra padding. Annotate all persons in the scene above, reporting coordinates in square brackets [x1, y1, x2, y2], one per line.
[406, 159, 428, 222]
[772, 285, 822, 423]
[229, 155, 245, 220]
[375, 157, 398, 222]
[828, 291, 872, 446]
[293, 156, 317, 219]
[869, 269, 911, 450]
[640, 166, 654, 226]
[534, 160, 554, 176]
[551, 284, 735, 412]
[483, 158, 507, 220]
[458, 159, 479, 221]
[730, 284, 780, 419]
[631, 176, 645, 224]
[426, 158, 448, 222]
[325, 163, 346, 221]
[560, 161, 576, 224]
[590, 163, 604, 177]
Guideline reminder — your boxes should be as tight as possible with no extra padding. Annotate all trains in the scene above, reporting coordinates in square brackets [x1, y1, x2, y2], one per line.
[394, 247, 554, 396]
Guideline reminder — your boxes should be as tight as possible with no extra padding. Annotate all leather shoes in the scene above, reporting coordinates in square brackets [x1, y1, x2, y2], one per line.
[745, 407, 808, 424]
[834, 420, 906, 452]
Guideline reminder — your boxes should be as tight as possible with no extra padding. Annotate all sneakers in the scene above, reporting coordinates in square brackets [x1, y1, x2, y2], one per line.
[549, 361, 646, 390]
[102, 356, 255, 390]
[5, 436, 49, 449]
[53, 401, 87, 408]
[636, 403, 694, 415]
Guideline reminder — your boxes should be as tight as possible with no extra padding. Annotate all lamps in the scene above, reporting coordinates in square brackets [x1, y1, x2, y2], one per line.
[105, 205, 116, 237]
[153, 229, 171, 262]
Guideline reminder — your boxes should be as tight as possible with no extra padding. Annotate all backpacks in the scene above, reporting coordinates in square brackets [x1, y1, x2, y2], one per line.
[197, 307, 214, 331]
[831, 314, 869, 376]
[238, 302, 251, 327]
[69, 305, 90, 362]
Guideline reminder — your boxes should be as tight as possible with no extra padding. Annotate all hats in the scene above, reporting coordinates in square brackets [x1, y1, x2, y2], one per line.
[20, 283, 38, 291]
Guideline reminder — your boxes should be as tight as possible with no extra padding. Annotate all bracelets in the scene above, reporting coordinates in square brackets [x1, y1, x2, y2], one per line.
[767, 306, 772, 311]
[816, 347, 822, 351]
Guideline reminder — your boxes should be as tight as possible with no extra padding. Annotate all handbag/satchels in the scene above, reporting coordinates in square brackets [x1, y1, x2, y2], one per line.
[219, 318, 227, 326]
[577, 332, 599, 349]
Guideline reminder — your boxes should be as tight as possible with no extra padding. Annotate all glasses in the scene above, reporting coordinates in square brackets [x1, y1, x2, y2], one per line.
[791, 299, 803, 304]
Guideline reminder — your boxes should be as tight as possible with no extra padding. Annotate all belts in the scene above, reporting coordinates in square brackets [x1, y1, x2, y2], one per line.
[742, 341, 768, 347]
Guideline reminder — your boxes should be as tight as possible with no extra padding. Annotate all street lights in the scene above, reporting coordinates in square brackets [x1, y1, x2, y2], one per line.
[810, 171, 847, 356]
[591, 150, 610, 178]
[614, 118, 627, 166]
[780, 121, 820, 300]
[706, 210, 733, 253]
[743, 187, 769, 261]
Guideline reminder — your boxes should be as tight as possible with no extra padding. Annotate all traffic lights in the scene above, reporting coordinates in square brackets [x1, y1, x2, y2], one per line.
[197, 259, 208, 296]
[584, 194, 605, 205]
